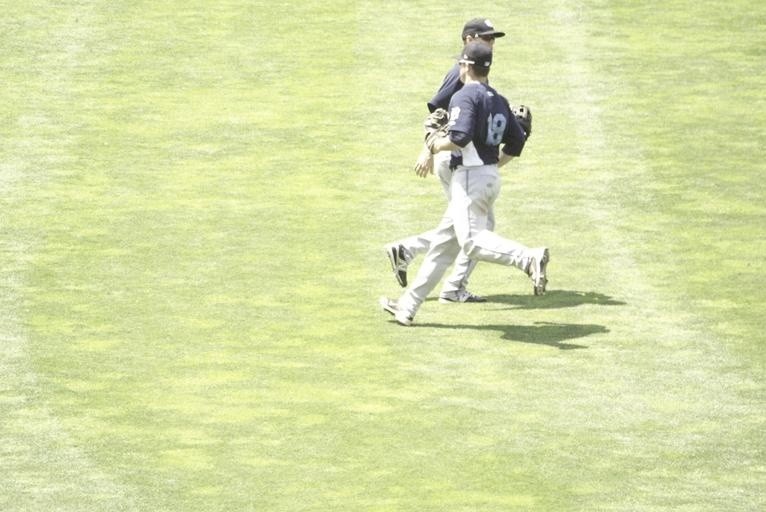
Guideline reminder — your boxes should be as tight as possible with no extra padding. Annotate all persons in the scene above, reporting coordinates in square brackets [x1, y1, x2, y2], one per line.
[385, 15, 506, 305]
[378, 36, 549, 327]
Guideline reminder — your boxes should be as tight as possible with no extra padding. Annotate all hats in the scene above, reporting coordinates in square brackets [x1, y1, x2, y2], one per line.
[454, 42, 492, 66]
[461, 16, 505, 41]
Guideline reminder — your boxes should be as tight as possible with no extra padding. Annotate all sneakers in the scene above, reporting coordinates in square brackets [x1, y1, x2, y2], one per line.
[385, 241, 410, 288]
[525, 247, 551, 297]
[439, 292, 487, 304]
[379, 294, 414, 327]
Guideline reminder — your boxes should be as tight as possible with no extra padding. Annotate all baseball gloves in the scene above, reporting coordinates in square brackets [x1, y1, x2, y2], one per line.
[425, 108, 449, 153]
[512, 106, 531, 140]
